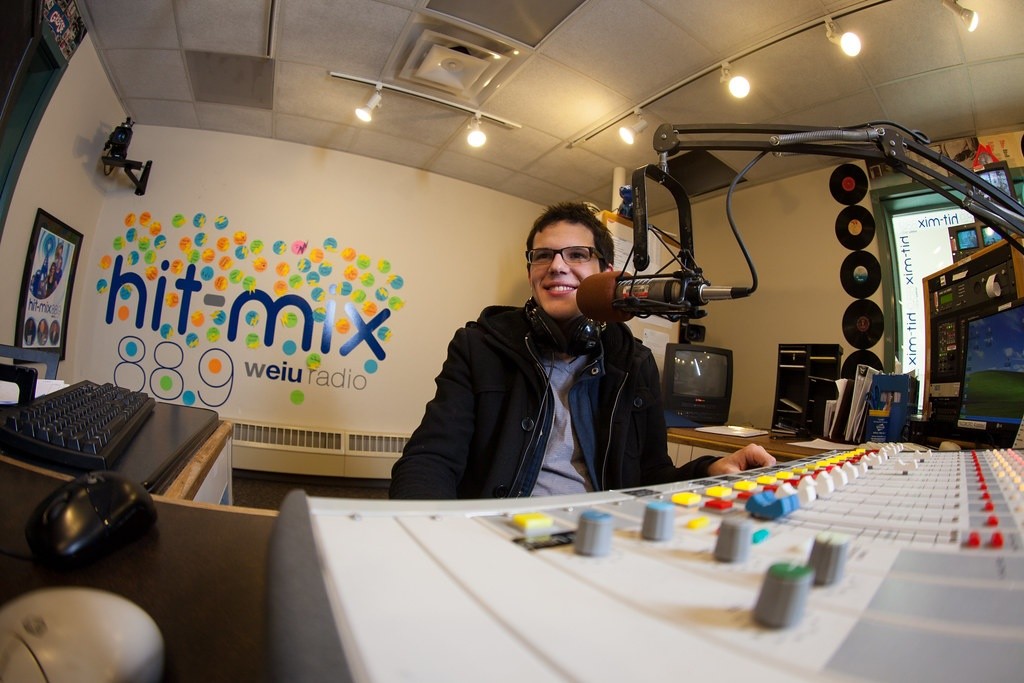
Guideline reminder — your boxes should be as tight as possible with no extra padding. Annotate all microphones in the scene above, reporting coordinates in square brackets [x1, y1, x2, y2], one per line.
[576, 270, 748, 323]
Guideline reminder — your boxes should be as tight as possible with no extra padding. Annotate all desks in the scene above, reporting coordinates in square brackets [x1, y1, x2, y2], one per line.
[667, 422, 949, 463]
[0, 416, 240, 502]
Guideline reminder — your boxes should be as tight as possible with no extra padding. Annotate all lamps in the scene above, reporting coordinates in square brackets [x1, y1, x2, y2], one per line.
[819, 17, 861, 56]
[940, 0, 980, 32]
[355, 84, 388, 123]
[467, 116, 485, 147]
[621, 109, 653, 144]
[720, 60, 753, 97]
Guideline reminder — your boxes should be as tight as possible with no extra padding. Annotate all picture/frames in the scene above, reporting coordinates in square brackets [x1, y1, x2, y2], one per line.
[14, 207, 84, 363]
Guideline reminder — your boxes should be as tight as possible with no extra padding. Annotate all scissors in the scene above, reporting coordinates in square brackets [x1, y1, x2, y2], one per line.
[883, 393, 893, 410]
[865, 384, 881, 410]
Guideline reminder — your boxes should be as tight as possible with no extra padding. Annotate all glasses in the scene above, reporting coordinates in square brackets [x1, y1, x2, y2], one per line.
[528, 246, 608, 271]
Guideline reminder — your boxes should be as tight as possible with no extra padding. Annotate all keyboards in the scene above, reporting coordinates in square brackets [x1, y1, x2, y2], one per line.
[0, 381, 154, 471]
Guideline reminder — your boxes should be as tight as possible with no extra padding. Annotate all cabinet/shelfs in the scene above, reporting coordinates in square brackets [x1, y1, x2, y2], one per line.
[771, 343, 843, 437]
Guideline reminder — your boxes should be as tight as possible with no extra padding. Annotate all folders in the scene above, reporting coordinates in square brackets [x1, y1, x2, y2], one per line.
[845, 364, 883, 440]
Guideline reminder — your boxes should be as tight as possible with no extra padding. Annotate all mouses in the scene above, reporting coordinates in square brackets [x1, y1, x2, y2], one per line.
[0, 586, 165, 683]
[25, 470, 158, 570]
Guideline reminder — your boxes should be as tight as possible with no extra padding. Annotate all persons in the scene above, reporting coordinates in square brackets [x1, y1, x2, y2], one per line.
[40, 255, 63, 295]
[388, 204, 775, 501]
[25, 320, 59, 345]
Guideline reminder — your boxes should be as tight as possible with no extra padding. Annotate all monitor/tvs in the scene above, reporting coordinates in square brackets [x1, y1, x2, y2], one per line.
[661, 343, 733, 423]
[922, 233, 1024, 452]
[0, 0, 68, 245]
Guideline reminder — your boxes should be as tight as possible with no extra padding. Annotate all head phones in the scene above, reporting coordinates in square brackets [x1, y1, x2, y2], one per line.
[525, 295, 607, 356]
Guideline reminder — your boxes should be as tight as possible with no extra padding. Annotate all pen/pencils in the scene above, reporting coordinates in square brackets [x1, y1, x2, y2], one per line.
[769, 435, 798, 439]
